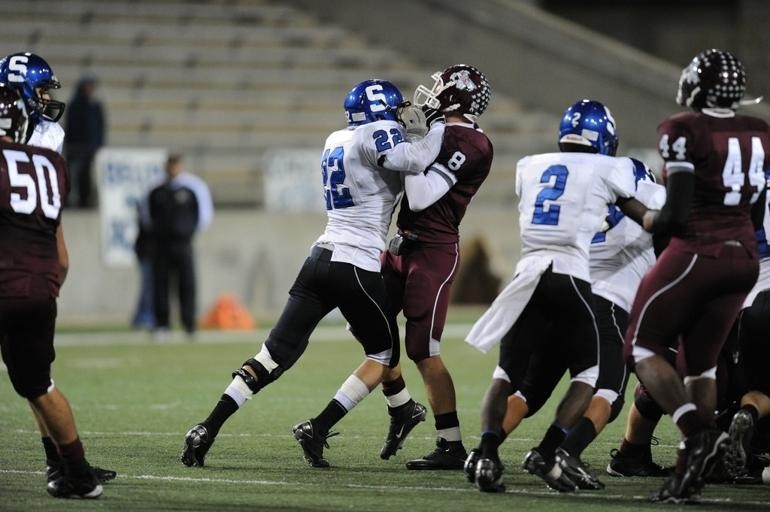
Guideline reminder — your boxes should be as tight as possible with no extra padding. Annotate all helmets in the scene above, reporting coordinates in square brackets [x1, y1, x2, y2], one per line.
[558, 99, 618, 156]
[675, 49, 746, 111]
[0, 52, 65, 144]
[344, 80, 410, 129]
[413, 64, 490, 122]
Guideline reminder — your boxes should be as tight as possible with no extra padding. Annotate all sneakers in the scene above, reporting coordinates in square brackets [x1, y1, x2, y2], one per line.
[48, 479, 104, 500]
[181, 425, 214, 467]
[474, 459, 509, 493]
[606, 447, 673, 479]
[291, 419, 339, 466]
[463, 450, 481, 479]
[380, 400, 426, 461]
[655, 408, 770, 503]
[48, 464, 115, 484]
[554, 447, 606, 490]
[406, 437, 468, 470]
[521, 448, 580, 493]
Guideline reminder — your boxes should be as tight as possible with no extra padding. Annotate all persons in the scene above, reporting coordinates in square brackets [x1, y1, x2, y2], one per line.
[467, 156, 669, 481]
[381, 64, 490, 472]
[604, 351, 730, 478]
[714, 223, 770, 485]
[139, 150, 205, 333]
[472, 100, 665, 494]
[0, 46, 116, 501]
[178, 73, 442, 467]
[60, 77, 110, 208]
[624, 44, 758, 498]
